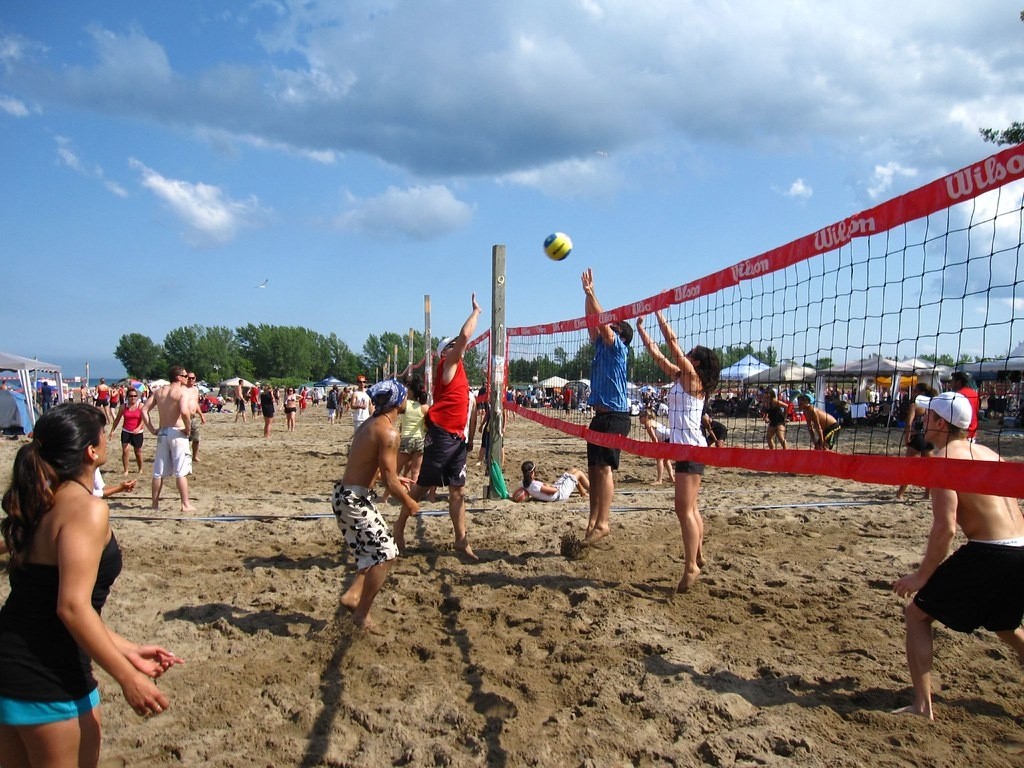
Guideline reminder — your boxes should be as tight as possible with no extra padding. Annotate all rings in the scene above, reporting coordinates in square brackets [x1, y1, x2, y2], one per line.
[153, 705, 161, 712]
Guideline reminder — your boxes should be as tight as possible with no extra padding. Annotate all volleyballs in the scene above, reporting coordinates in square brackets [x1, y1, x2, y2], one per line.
[542, 231, 574, 261]
[512, 488, 530, 502]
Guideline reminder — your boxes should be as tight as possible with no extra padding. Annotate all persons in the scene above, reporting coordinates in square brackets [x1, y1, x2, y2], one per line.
[332, 380, 421, 636]
[636, 287, 720, 594]
[891, 392, 1024, 723]
[142, 366, 196, 512]
[579, 267, 633, 548]
[0, 372, 1023, 502]
[393, 291, 482, 561]
[0, 402, 184, 768]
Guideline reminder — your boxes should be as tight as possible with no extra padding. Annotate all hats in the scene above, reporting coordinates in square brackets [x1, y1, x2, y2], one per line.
[357, 376, 366, 381]
[915, 392, 972, 430]
[436, 337, 458, 359]
[366, 380, 408, 418]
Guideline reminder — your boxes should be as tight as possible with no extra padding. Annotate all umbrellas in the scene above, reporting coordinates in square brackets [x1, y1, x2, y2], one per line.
[131, 379, 147, 393]
[641, 385, 659, 393]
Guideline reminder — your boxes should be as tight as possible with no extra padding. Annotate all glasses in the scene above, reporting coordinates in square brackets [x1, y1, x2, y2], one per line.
[359, 381, 366, 383]
[189, 377, 195, 379]
[443, 343, 455, 351]
[288, 390, 293, 392]
[180, 375, 188, 378]
[128, 395, 138, 398]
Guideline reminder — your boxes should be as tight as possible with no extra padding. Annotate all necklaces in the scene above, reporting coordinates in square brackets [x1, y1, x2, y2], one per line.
[67, 478, 92, 495]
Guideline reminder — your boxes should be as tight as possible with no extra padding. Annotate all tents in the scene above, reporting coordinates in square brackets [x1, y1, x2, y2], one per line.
[718, 354, 772, 381]
[817, 355, 1024, 386]
[741, 362, 816, 384]
[313, 376, 350, 405]
[535, 376, 570, 388]
[0, 352, 64, 430]
[220, 375, 256, 400]
